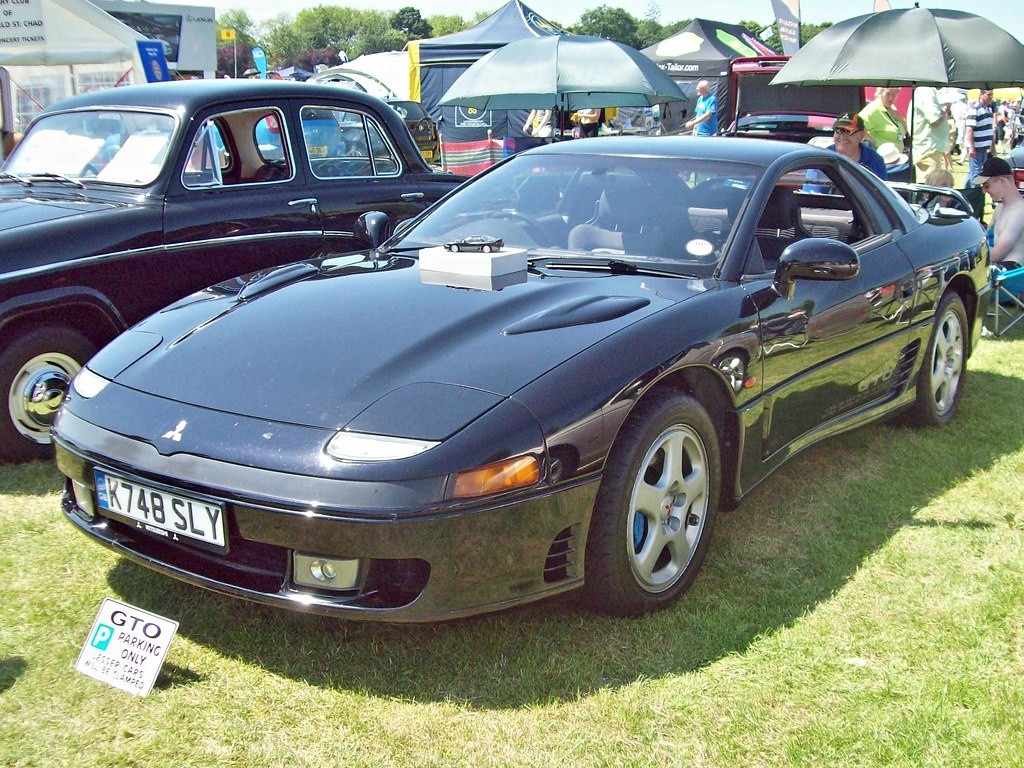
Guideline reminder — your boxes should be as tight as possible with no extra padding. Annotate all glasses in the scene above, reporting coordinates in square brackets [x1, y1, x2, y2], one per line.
[836, 128, 858, 136]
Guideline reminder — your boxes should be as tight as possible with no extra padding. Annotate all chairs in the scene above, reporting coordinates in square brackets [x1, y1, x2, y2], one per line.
[988, 226, 1024, 336]
[569, 186, 661, 254]
[727, 187, 812, 269]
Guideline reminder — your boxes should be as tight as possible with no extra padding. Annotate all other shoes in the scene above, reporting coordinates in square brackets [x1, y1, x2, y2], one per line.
[949, 168, 953, 173]
[956, 161, 964, 166]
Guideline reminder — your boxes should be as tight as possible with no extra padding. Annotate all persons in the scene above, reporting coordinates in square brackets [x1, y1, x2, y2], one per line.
[802, 112, 888, 199]
[684, 79, 718, 136]
[982, 156, 1024, 302]
[523, 107, 601, 141]
[855, 83, 1024, 208]
[1, 129, 25, 159]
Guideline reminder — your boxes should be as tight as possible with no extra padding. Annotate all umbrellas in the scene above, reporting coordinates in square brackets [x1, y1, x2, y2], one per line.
[436, 32, 692, 141]
[768, 1, 1024, 206]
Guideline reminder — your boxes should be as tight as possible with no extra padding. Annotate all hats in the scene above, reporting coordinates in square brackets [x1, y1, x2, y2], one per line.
[971, 157, 1013, 185]
[876, 142, 909, 166]
[832, 112, 865, 132]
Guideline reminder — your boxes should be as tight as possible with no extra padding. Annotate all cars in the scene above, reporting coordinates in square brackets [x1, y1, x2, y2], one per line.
[49, 135, 992, 627]
[712, 55, 916, 188]
[331, 99, 438, 164]
[208, 108, 346, 162]
[1003, 140, 1024, 200]
[0, 76, 524, 461]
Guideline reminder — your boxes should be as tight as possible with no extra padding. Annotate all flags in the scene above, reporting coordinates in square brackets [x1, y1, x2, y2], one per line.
[221, 28, 236, 40]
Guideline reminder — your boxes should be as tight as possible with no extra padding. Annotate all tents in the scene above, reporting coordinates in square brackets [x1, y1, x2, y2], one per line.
[407, 0, 576, 160]
[637, 18, 784, 137]
[0, 0, 152, 154]
